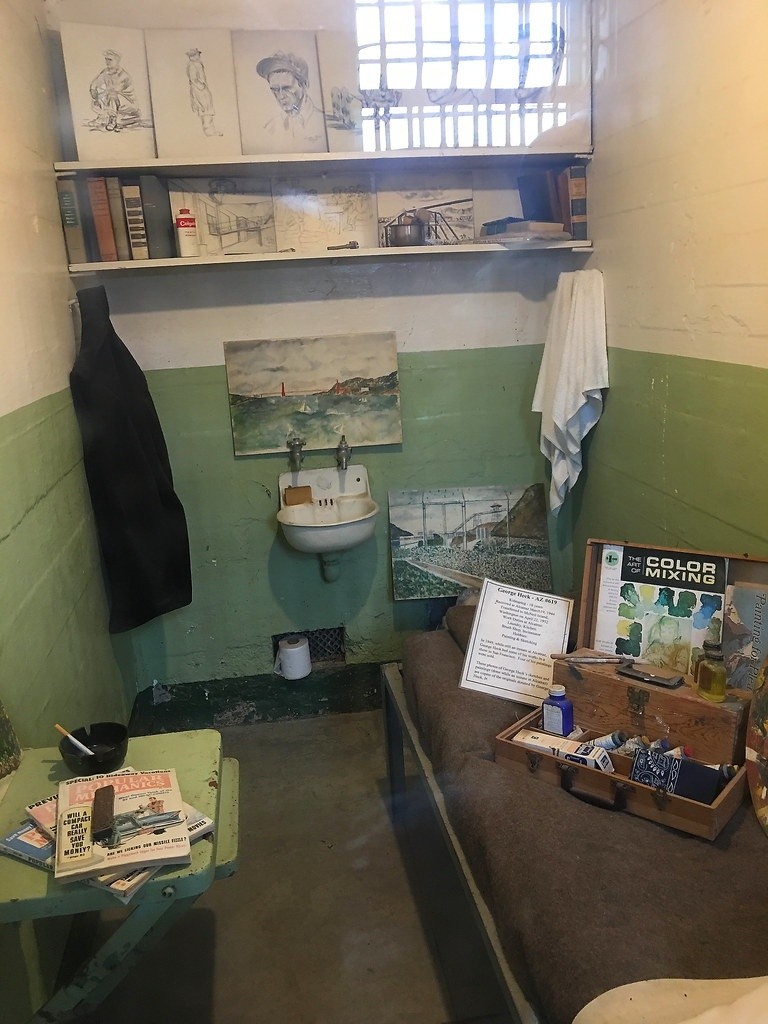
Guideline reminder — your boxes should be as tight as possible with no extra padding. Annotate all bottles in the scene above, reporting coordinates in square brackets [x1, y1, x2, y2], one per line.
[703, 763, 736, 780]
[663, 745, 692, 759]
[542, 685, 573, 737]
[648, 738, 670, 753]
[618, 736, 651, 751]
[584, 731, 626, 750]
[694, 640, 722, 684]
[696, 651, 727, 703]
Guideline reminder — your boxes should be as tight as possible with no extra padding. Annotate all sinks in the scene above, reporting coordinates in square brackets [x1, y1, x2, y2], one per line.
[275, 465, 379, 553]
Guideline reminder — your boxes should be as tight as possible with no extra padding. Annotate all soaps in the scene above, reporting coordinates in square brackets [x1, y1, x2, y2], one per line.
[285, 486, 313, 506]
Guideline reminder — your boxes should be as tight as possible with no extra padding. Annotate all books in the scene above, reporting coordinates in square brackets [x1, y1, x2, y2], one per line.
[0, 766, 214, 896]
[517, 166, 587, 240]
[56, 174, 175, 263]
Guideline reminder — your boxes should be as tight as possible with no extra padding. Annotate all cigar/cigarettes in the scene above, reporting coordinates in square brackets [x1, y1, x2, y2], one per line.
[55, 724, 94, 755]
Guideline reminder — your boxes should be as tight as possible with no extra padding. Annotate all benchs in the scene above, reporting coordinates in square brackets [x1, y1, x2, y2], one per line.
[375, 605, 768, 1024]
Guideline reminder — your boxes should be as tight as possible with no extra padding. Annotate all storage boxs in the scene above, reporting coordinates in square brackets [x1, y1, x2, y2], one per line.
[511, 728, 616, 773]
[552, 647, 752, 763]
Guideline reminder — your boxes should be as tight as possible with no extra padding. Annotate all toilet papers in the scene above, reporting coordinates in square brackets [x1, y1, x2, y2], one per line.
[274, 637, 312, 679]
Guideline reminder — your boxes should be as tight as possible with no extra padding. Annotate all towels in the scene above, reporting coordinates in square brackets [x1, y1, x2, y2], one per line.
[529, 270, 608, 518]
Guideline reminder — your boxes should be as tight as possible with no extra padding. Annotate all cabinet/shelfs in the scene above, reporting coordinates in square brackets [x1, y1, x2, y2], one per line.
[53, 147, 597, 272]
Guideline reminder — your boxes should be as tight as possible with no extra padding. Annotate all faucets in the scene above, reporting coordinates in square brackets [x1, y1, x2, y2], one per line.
[287, 436, 307, 470]
[337, 434, 352, 471]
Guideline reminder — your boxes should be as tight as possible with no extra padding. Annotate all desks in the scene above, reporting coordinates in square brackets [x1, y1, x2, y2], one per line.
[0, 729, 241, 1024]
